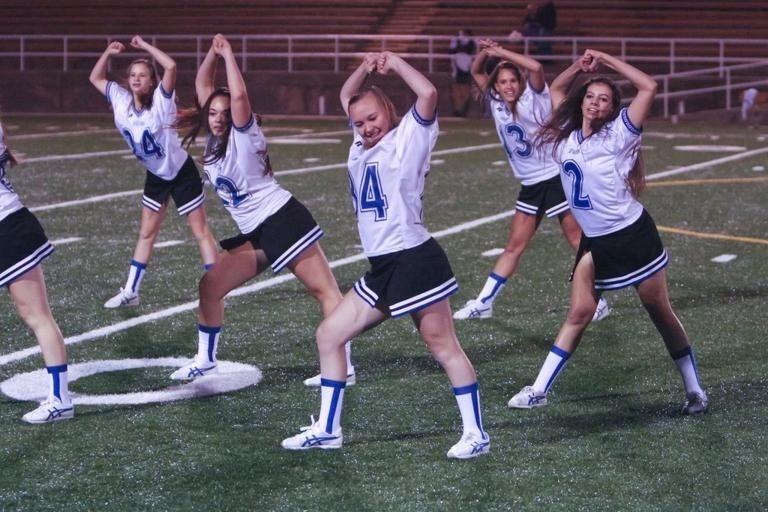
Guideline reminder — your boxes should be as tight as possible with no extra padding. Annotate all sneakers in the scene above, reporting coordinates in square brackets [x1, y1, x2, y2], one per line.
[591, 298, 609, 321]
[24, 398, 77, 427]
[447, 433, 490, 459]
[281, 422, 343, 450]
[303, 366, 356, 386]
[508, 385, 548, 408]
[452, 300, 493, 324]
[104, 289, 139, 309]
[680, 391, 709, 416]
[169, 361, 219, 380]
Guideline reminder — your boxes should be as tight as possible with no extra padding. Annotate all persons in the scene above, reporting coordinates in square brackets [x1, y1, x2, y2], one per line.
[535, 0, 557, 53]
[741, 88, 759, 121]
[506, 49, 708, 415]
[452, 38, 609, 321]
[169, 33, 355, 387]
[0, 125, 74, 425]
[281, 51, 490, 459]
[521, 5, 540, 37]
[89, 35, 219, 309]
